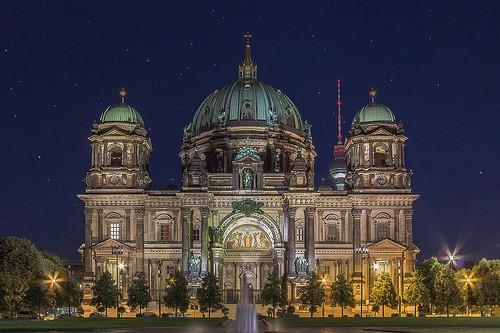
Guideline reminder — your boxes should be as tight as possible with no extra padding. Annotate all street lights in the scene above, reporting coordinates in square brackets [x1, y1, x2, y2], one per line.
[361, 256, 379, 318]
[110, 245, 123, 317]
